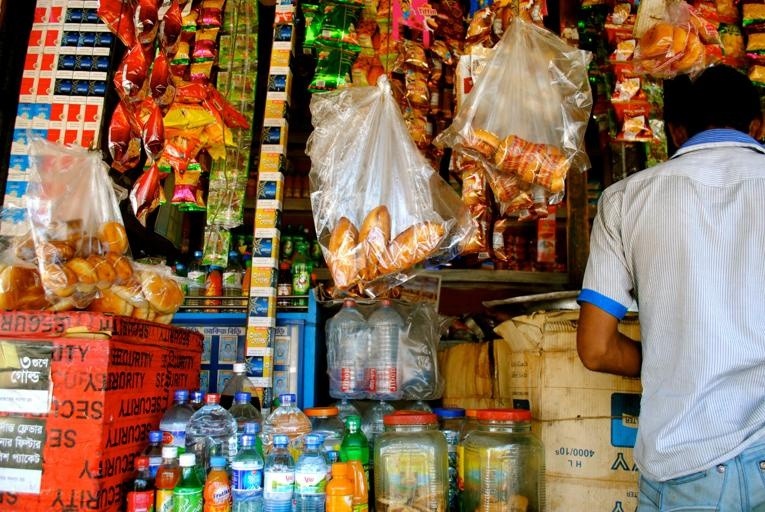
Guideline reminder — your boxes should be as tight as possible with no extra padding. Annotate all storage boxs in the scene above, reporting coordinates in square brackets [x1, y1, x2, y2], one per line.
[494, 306, 641, 512]
[0, 310, 204, 512]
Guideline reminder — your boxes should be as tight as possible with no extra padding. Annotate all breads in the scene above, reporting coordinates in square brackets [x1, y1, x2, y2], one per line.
[464, 129, 571, 194]
[637, 22, 701, 73]
[378, 222, 443, 274]
[356, 206, 392, 280]
[0, 221, 184, 325]
[329, 214, 358, 289]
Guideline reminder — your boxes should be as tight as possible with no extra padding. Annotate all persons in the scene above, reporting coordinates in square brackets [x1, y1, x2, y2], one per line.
[576, 64, 765, 512]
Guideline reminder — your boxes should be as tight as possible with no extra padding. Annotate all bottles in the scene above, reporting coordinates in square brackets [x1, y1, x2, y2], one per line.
[133, 155, 325, 312]
[326, 299, 367, 399]
[121, 363, 547, 512]
[367, 299, 406, 399]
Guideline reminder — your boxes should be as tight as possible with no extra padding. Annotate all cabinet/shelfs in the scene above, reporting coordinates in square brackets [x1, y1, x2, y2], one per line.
[244, 197, 568, 353]
